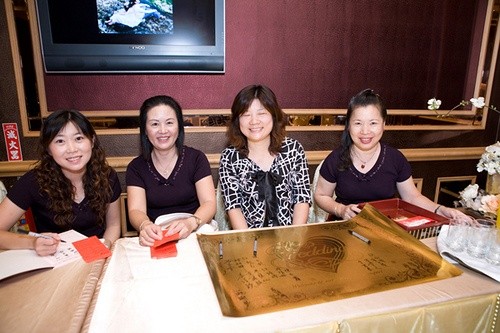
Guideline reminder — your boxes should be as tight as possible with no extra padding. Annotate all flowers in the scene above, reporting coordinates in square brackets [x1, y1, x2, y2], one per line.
[454, 182, 499, 216]
[475, 140, 500, 176]
[428, 96, 500, 121]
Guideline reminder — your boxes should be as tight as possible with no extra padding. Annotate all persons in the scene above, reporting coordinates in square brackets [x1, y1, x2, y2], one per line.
[125, 95, 216, 246]
[0, 109, 122, 257]
[318, 89, 479, 226]
[219, 85, 313, 230]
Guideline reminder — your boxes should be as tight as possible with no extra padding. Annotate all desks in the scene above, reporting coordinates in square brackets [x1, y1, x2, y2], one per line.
[0, 109, 500, 332]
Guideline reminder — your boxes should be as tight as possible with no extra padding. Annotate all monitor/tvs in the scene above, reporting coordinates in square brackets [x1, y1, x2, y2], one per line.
[35, 0, 226, 75]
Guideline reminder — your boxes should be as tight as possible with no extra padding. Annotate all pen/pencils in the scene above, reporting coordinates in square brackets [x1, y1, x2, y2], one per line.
[348, 229, 371, 244]
[16, 228, 66, 244]
[253, 237, 257, 256]
[219, 241, 223, 259]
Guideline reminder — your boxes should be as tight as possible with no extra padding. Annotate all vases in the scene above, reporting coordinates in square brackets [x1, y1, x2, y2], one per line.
[485, 172, 500, 196]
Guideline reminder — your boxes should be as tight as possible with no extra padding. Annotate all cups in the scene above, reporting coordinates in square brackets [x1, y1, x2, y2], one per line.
[445, 217, 500, 266]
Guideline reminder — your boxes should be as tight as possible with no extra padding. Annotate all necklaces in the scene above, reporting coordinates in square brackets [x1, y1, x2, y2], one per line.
[351, 144, 380, 168]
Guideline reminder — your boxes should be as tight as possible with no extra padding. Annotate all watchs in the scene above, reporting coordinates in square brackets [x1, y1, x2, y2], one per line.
[192, 216, 201, 232]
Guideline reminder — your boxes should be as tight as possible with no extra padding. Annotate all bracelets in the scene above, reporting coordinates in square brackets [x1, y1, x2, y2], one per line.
[434, 204, 442, 214]
